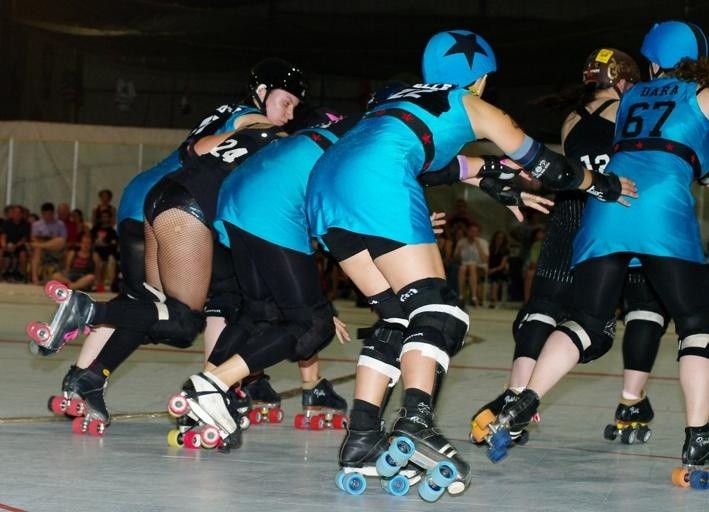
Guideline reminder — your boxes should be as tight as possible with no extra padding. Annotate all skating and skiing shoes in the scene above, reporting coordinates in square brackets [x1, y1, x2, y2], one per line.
[672, 422, 709, 489]
[26, 280, 97, 357]
[48, 365, 111, 437]
[605, 389, 654, 445]
[167, 373, 252, 454]
[247, 380, 283, 424]
[469, 384, 541, 464]
[295, 376, 348, 430]
[376, 396, 471, 503]
[335, 419, 424, 496]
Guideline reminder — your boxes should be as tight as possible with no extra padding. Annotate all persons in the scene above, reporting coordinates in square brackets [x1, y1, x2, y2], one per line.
[244, 353, 348, 412]
[305, 30, 638, 503]
[471, 19, 708, 488]
[434, 199, 548, 311]
[467, 49, 669, 446]
[1, 188, 119, 292]
[32, 112, 352, 428]
[49, 59, 306, 430]
[311, 237, 359, 300]
[178, 81, 556, 452]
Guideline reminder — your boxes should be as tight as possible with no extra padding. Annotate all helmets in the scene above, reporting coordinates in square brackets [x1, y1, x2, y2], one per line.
[422, 30, 497, 88]
[582, 47, 647, 91]
[641, 21, 709, 69]
[245, 57, 307, 100]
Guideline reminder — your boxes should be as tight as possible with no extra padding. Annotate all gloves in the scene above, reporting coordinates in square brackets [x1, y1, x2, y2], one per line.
[477, 155, 524, 180]
[584, 170, 621, 202]
[480, 176, 526, 208]
